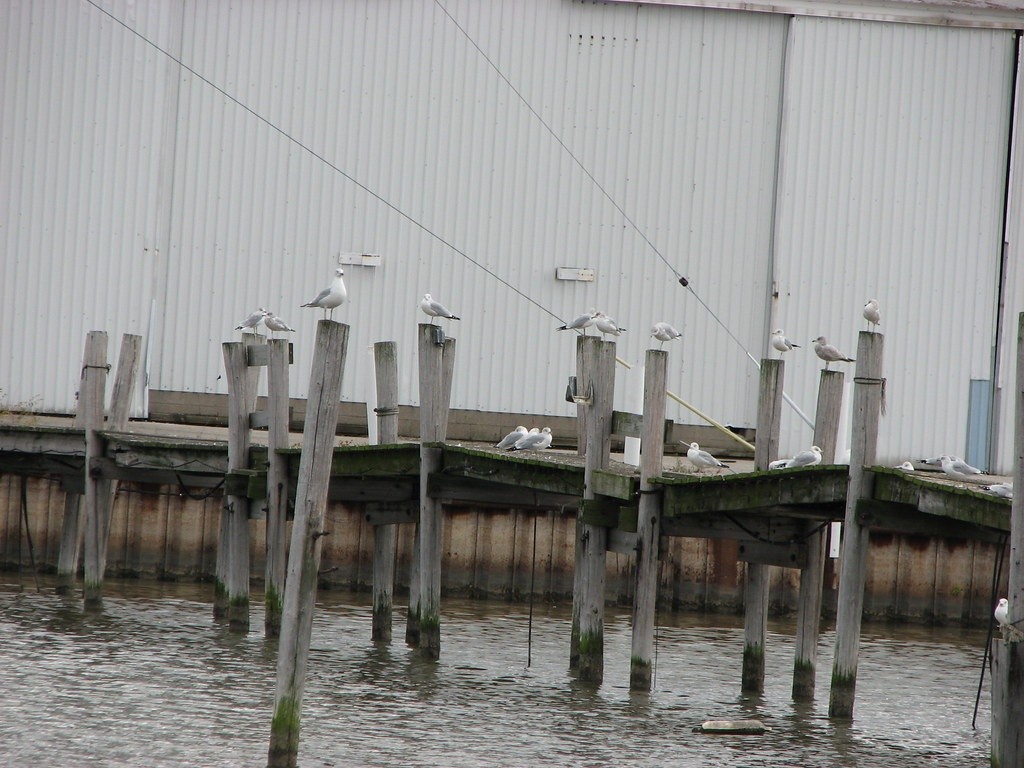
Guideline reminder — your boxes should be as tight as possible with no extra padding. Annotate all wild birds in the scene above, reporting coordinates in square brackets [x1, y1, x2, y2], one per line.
[937, 456, 981, 479]
[895, 461, 914, 471]
[772, 328, 802, 359]
[785, 446, 824, 466]
[811, 336, 856, 370]
[917, 457, 943, 468]
[863, 299, 881, 334]
[994, 598, 1009, 626]
[234, 307, 266, 337]
[687, 442, 730, 475]
[650, 322, 682, 350]
[262, 312, 296, 339]
[421, 294, 461, 324]
[556, 311, 627, 341]
[300, 268, 347, 320]
[493, 426, 553, 454]
[990, 482, 1013, 498]
